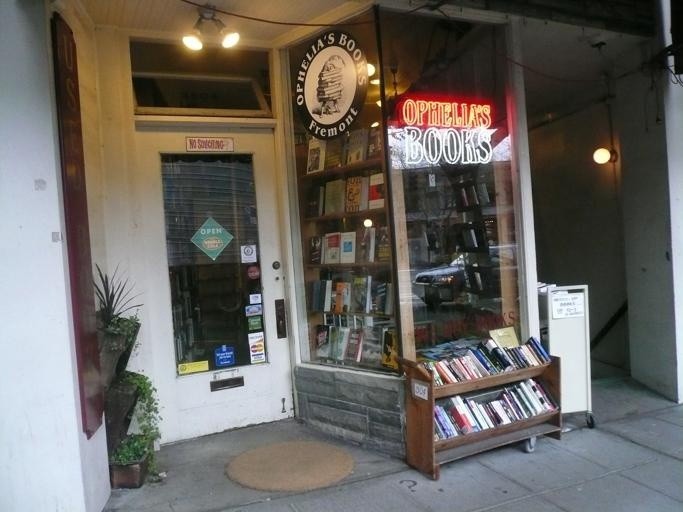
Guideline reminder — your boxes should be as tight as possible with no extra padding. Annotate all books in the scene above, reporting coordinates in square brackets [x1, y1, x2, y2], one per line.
[413, 325, 561, 443]
[292, 122, 399, 375]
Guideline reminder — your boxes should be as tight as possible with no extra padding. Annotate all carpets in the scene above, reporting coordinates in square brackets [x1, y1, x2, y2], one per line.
[224, 441, 354, 491]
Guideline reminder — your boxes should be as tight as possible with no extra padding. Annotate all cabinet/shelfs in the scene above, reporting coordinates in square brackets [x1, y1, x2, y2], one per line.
[302, 159, 392, 317]
[396, 351, 560, 481]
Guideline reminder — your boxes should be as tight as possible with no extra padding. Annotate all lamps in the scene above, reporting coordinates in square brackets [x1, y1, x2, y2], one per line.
[182, 4, 241, 51]
[593, 147, 618, 164]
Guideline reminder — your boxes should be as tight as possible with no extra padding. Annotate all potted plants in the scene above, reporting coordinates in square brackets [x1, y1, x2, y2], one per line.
[92, 259, 163, 488]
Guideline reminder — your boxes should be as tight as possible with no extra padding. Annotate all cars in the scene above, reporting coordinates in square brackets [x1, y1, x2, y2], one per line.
[413, 245, 518, 307]
